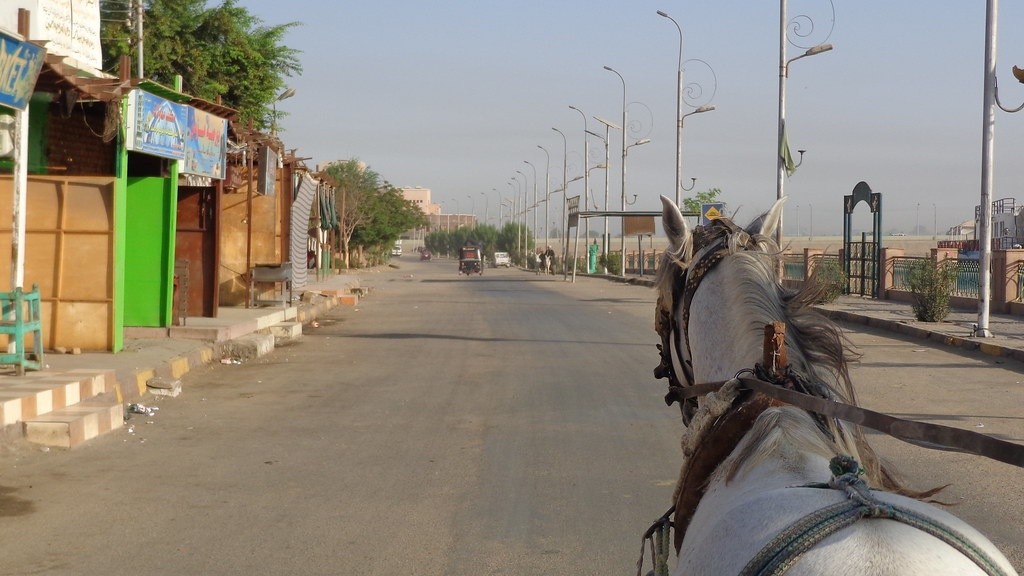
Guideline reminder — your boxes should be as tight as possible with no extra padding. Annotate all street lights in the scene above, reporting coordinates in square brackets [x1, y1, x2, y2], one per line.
[553, 126, 585, 274]
[604, 65, 652, 279]
[656, 9, 717, 215]
[776, 44, 835, 284]
[973, 66, 1024, 340]
[536, 144, 563, 273]
[440, 161, 546, 271]
[570, 104, 606, 272]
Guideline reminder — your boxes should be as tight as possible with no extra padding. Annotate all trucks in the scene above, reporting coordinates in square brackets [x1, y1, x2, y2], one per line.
[458, 246, 483, 277]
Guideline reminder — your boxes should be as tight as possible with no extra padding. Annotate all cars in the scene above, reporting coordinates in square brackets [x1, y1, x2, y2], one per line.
[491, 252, 511, 269]
[392, 246, 402, 256]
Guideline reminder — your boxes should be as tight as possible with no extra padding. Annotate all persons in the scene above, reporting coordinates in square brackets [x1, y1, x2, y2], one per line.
[536, 245, 554, 262]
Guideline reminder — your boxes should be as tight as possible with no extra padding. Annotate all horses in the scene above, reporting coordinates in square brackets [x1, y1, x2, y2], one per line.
[533, 252, 552, 276]
[654, 194, 1024, 576]
